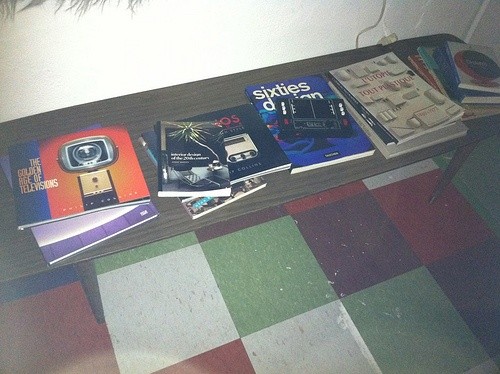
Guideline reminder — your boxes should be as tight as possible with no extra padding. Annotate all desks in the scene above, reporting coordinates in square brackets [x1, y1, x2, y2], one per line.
[0, 33, 500, 324]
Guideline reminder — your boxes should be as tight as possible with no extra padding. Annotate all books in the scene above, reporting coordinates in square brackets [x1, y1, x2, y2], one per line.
[0, 39, 500, 269]
